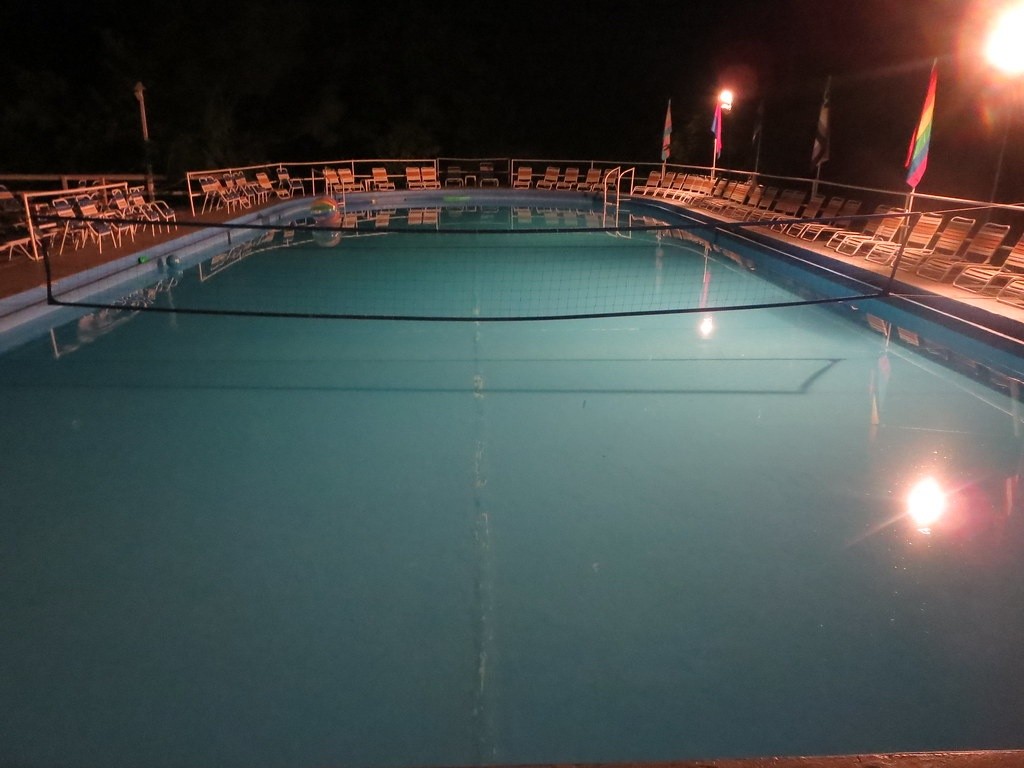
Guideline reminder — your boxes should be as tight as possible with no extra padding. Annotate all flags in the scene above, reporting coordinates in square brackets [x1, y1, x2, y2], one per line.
[752, 122, 763, 146]
[662, 99, 673, 161]
[904, 57, 939, 188]
[811, 77, 832, 170]
[711, 94, 723, 158]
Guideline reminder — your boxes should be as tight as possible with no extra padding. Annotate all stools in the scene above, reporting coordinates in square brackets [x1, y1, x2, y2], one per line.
[465, 175, 477, 188]
[360, 179, 377, 192]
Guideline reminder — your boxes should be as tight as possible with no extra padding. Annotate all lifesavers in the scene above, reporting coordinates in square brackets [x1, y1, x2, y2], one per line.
[443, 195, 470, 203]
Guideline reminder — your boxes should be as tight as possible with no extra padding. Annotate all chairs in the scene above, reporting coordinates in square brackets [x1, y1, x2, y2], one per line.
[591, 168, 617, 194]
[915, 222, 1010, 283]
[0, 178, 177, 261]
[322, 168, 351, 199]
[800, 195, 846, 241]
[445, 166, 464, 188]
[835, 207, 909, 257]
[480, 162, 499, 188]
[631, 171, 662, 196]
[406, 167, 426, 190]
[576, 169, 602, 193]
[196, 207, 756, 288]
[651, 171, 805, 242]
[536, 166, 560, 191]
[952, 232, 1024, 295]
[513, 167, 533, 189]
[786, 194, 826, 238]
[891, 215, 976, 272]
[338, 168, 366, 193]
[556, 167, 580, 192]
[995, 276, 1024, 309]
[855, 311, 1024, 399]
[799, 197, 862, 243]
[198, 168, 306, 215]
[865, 211, 944, 264]
[372, 167, 396, 192]
[823, 204, 892, 252]
[421, 166, 441, 190]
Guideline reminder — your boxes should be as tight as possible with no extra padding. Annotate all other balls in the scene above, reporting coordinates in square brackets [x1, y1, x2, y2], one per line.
[309, 196, 340, 224]
[311, 229, 342, 247]
